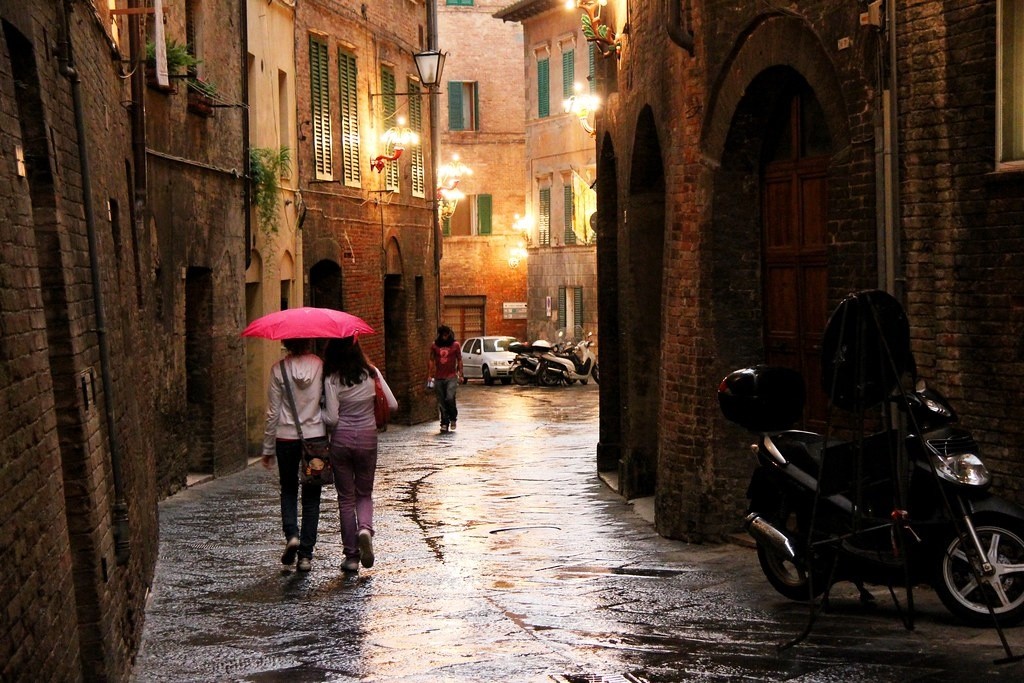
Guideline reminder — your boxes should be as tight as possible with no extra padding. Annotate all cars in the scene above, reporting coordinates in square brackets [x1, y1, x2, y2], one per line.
[458, 337, 531, 385]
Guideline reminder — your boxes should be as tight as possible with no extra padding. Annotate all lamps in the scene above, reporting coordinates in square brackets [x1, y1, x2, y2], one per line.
[370, 49, 450, 95]
[566, 0, 621, 57]
[563, 83, 600, 138]
[370, 116, 418, 172]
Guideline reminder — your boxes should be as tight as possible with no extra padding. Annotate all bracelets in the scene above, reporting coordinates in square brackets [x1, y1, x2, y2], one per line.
[459, 375, 463, 377]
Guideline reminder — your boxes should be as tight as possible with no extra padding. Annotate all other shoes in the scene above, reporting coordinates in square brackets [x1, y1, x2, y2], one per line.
[358, 529, 374, 568]
[299, 557, 311, 571]
[451, 422, 456, 429]
[281, 536, 299, 565]
[340, 560, 358, 570]
[440, 425, 448, 432]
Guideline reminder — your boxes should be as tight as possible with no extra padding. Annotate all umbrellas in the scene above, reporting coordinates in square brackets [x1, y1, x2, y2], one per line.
[240, 308, 376, 344]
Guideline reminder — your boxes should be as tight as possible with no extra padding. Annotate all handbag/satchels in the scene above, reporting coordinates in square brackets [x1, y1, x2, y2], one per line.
[371, 364, 390, 428]
[300, 439, 333, 484]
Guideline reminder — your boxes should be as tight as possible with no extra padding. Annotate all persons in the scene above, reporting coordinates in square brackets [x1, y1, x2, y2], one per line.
[428, 325, 465, 432]
[321, 337, 399, 572]
[261, 339, 328, 573]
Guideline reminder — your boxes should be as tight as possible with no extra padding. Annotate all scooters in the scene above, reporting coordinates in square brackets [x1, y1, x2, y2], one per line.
[533, 327, 602, 388]
[508, 329, 591, 386]
[714, 286, 1024, 625]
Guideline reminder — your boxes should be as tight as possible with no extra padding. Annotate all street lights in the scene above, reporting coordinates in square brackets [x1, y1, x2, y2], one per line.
[413, 44, 448, 441]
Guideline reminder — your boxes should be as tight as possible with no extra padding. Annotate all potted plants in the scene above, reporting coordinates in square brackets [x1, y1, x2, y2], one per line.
[187, 77, 217, 115]
[147, 31, 204, 92]
